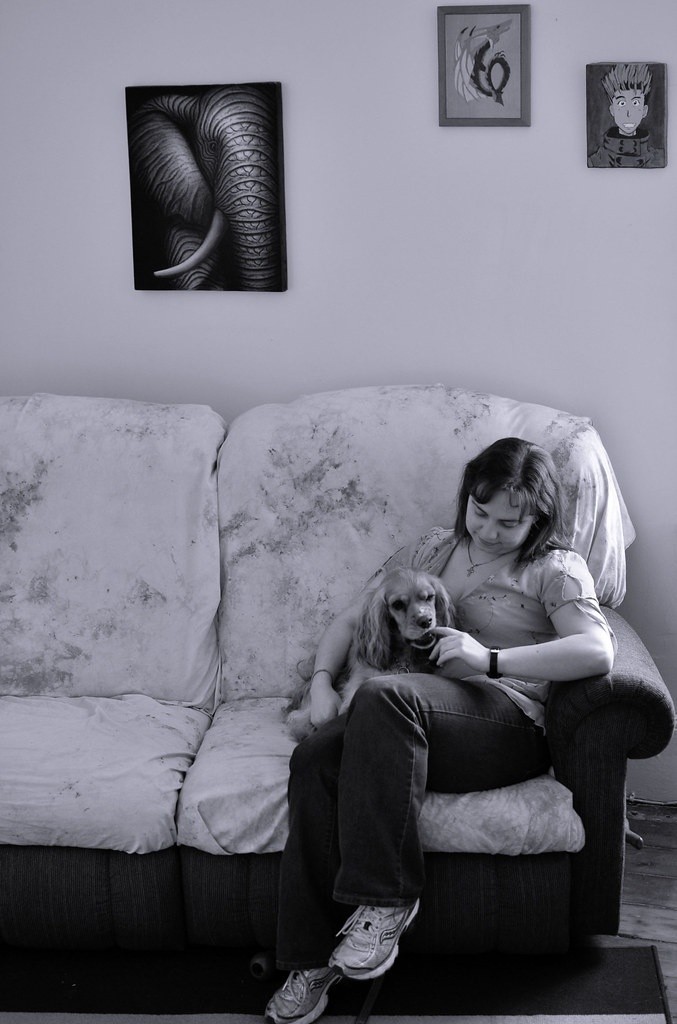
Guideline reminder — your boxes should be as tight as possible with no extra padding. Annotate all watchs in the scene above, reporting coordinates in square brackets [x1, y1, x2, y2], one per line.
[487, 647, 503, 679]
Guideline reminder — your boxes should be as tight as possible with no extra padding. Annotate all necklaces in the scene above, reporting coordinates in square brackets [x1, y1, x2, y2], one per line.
[467, 538, 504, 576]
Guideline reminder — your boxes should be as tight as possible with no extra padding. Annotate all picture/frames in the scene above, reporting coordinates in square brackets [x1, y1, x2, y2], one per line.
[435, 5, 534, 128]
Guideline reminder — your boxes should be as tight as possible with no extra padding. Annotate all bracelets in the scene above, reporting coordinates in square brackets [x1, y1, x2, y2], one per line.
[310, 669, 333, 687]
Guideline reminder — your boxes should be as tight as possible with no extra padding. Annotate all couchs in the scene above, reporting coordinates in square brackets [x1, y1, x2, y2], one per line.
[2, 383, 673, 1016]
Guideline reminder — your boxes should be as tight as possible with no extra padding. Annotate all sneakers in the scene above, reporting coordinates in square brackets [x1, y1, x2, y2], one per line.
[328, 893, 421, 980]
[265, 965, 343, 1024]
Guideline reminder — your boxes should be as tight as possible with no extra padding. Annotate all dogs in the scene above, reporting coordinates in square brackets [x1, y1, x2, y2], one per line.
[280, 568, 456, 742]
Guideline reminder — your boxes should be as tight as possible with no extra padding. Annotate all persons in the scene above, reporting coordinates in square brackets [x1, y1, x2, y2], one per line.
[264, 436, 618, 1024]
